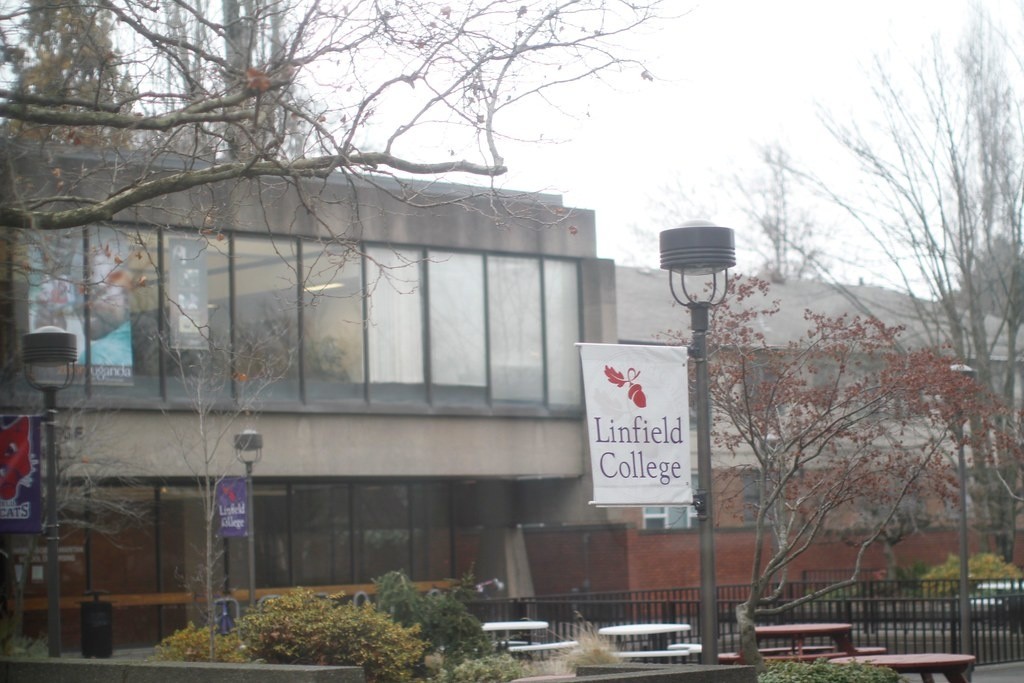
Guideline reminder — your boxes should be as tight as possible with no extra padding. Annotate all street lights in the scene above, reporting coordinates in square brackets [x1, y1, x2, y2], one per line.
[21, 325, 77, 658]
[659, 220, 736, 665]
[949, 364, 978, 683]
[234, 429, 263, 606]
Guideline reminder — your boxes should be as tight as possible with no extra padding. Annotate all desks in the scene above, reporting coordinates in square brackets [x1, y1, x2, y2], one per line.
[827, 653, 976, 683]
[598, 623, 691, 664]
[755, 623, 855, 652]
[482, 620, 549, 660]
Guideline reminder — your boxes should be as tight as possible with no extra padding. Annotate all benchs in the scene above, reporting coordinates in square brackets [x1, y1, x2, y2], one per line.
[496, 641, 541, 653]
[668, 644, 703, 664]
[611, 648, 691, 664]
[507, 641, 580, 661]
[717, 645, 886, 665]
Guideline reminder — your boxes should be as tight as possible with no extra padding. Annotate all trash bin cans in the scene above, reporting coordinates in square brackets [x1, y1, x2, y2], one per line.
[79, 590, 113, 659]
